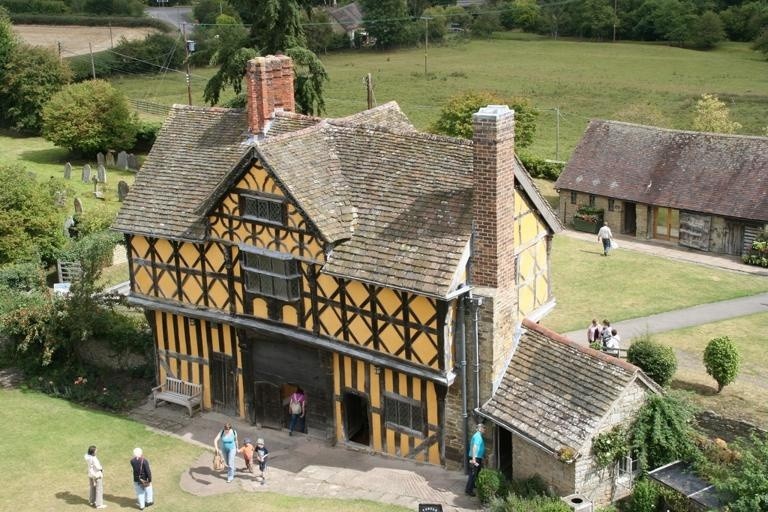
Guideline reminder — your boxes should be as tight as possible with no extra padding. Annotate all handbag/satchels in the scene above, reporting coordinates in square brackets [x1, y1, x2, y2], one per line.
[139, 478, 150, 488]
[212, 455, 225, 471]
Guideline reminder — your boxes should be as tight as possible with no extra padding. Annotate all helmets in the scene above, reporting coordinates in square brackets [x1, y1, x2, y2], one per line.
[256, 439, 264, 445]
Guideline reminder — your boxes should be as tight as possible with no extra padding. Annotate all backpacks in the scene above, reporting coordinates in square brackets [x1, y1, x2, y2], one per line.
[290, 401, 302, 414]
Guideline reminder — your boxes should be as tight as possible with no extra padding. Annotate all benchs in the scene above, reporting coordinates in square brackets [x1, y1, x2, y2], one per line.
[151, 377, 203, 417]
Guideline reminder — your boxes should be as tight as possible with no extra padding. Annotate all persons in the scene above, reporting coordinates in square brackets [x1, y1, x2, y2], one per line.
[603, 329, 619, 365]
[586, 318, 601, 344]
[600, 319, 612, 351]
[596, 221, 613, 257]
[83, 444, 107, 510]
[463, 422, 487, 498]
[128, 447, 154, 511]
[212, 422, 239, 484]
[237, 438, 254, 475]
[252, 438, 270, 486]
[287, 388, 305, 437]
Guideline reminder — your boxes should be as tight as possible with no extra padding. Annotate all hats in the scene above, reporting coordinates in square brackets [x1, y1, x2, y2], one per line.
[243, 439, 251, 444]
